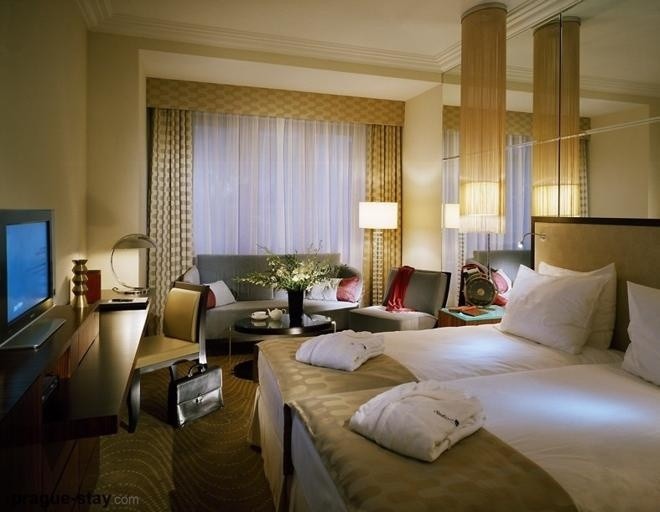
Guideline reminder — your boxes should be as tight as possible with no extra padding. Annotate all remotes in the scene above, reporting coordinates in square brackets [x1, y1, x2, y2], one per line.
[112, 297, 134, 302]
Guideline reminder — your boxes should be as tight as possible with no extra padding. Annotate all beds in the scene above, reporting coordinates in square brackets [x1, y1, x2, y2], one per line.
[246, 215, 660, 512]
[276, 360, 660, 512]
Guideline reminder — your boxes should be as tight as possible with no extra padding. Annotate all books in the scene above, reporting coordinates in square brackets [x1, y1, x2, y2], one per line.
[449, 304, 488, 317]
[97, 297, 149, 310]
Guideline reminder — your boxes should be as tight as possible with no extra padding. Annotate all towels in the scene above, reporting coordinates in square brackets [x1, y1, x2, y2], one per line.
[387, 266, 416, 311]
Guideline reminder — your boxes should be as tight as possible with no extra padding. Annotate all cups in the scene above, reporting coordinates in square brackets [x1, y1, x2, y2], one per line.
[251, 312, 266, 317]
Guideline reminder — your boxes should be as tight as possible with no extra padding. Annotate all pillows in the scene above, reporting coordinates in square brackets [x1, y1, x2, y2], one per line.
[538, 260, 617, 351]
[490, 264, 608, 355]
[306, 278, 342, 301]
[336, 274, 359, 303]
[622, 280, 660, 386]
[200, 284, 236, 308]
[203, 280, 236, 307]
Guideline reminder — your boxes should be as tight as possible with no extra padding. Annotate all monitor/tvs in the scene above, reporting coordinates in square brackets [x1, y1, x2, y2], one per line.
[0, 208, 69, 351]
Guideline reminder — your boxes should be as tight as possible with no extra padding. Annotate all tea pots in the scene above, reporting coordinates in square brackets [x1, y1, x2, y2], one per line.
[267, 308, 286, 320]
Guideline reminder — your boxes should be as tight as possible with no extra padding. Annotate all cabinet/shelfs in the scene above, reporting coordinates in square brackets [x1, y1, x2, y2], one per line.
[0, 305, 118, 512]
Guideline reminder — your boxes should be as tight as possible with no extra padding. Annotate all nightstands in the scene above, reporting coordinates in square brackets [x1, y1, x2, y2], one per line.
[438, 304, 504, 325]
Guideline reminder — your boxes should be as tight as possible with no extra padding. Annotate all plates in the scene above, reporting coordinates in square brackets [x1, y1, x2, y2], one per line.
[251, 315, 268, 320]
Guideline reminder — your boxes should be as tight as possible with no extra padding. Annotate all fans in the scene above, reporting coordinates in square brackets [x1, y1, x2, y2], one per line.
[463, 275, 498, 307]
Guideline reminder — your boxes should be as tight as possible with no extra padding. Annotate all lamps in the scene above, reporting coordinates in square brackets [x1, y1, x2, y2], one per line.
[444, 204, 480, 306]
[359, 202, 397, 306]
[518, 231, 547, 248]
[110, 235, 157, 295]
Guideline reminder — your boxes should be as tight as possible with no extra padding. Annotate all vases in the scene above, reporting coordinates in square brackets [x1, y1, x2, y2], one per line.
[287, 287, 306, 320]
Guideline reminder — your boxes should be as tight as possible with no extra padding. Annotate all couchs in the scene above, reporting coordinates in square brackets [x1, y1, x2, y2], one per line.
[348, 266, 451, 331]
[183, 253, 363, 339]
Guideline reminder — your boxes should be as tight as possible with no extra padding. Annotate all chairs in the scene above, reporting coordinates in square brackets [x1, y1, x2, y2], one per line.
[126, 281, 209, 434]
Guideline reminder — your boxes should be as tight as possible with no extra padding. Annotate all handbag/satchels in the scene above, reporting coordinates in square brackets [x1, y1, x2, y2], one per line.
[168, 364, 224, 428]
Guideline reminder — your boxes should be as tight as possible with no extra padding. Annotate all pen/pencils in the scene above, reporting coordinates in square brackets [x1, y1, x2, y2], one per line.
[112, 299, 133, 302]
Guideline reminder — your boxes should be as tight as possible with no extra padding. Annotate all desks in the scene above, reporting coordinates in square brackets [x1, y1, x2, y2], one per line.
[90, 288, 151, 435]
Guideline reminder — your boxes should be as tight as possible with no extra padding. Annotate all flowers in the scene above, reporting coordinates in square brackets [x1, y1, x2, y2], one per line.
[230, 240, 351, 292]
